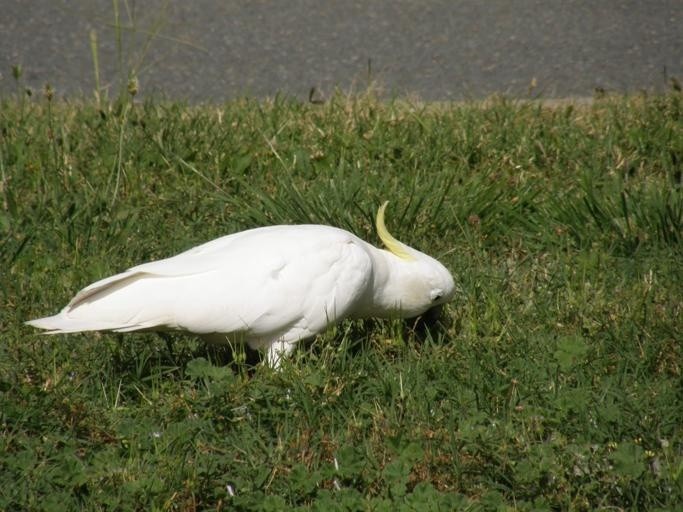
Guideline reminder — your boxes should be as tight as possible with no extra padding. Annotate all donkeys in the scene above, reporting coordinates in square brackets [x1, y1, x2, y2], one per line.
[24, 201, 455, 376]
